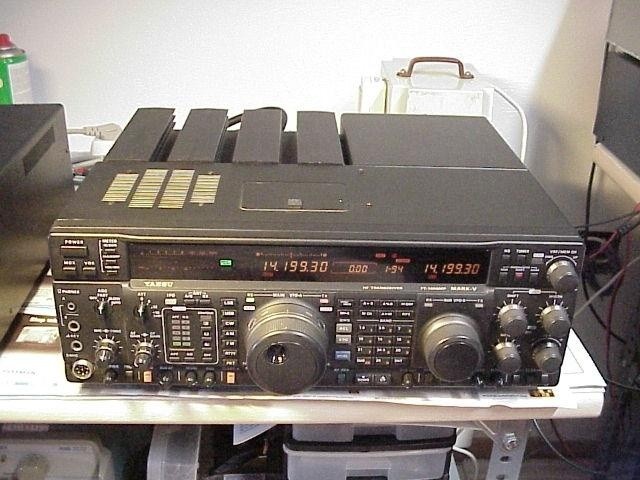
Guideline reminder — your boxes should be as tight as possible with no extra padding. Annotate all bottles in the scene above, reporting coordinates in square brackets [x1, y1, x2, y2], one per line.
[0, 33, 34, 104]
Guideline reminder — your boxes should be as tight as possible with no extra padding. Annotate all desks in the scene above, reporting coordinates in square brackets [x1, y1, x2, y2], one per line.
[0, 264, 605, 479]
